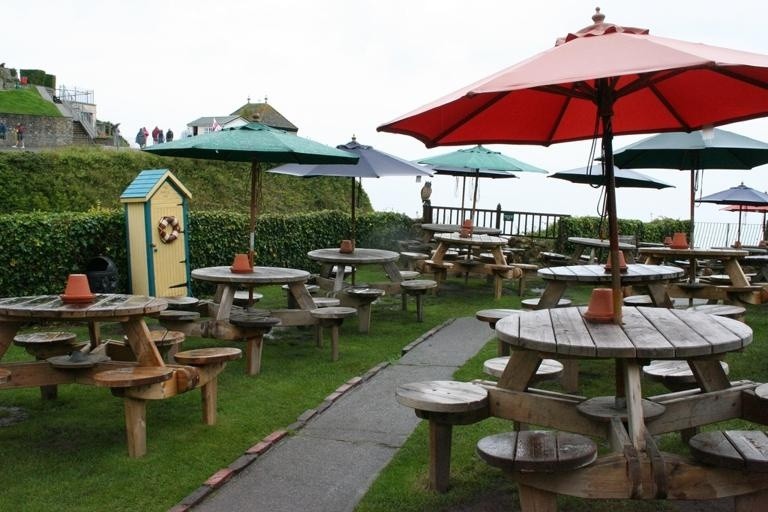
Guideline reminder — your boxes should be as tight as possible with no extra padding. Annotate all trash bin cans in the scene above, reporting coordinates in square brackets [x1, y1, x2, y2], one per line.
[87, 256, 118, 292]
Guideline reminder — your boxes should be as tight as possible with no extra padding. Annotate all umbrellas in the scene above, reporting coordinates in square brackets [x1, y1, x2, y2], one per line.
[409, 141, 549, 292]
[265, 135, 435, 285]
[429, 166, 519, 223]
[374, 7, 768, 408]
[593, 125, 767, 306]
[693, 180, 767, 248]
[546, 160, 675, 263]
[720, 202, 768, 241]
[142, 114, 360, 307]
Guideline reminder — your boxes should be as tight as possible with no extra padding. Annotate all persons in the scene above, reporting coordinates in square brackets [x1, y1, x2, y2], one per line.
[0, 120, 26, 149]
[111, 123, 173, 152]
